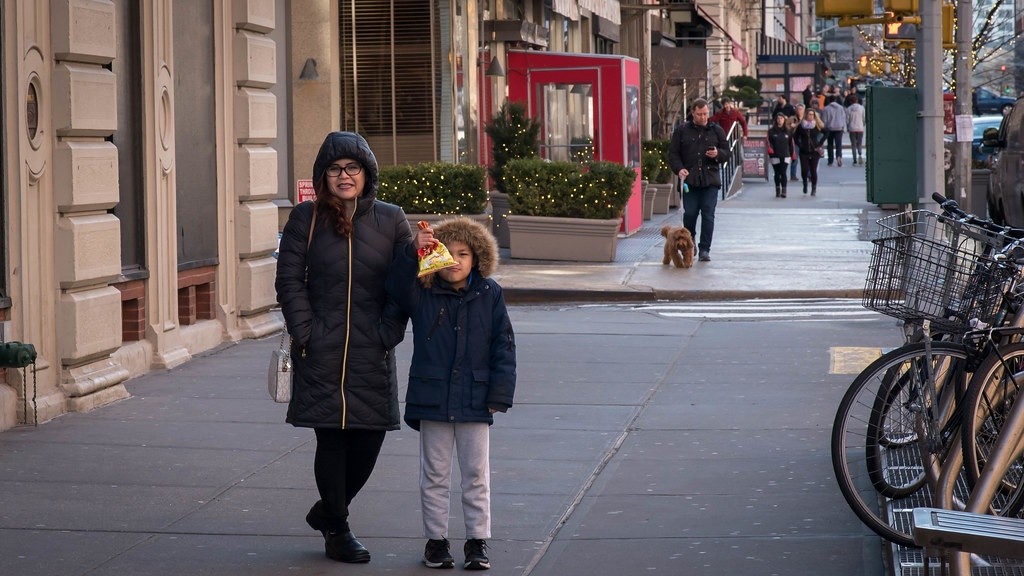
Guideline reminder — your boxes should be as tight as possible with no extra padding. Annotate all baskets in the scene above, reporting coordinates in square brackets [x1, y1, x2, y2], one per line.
[867, 235, 1022, 338]
[876, 209, 1022, 268]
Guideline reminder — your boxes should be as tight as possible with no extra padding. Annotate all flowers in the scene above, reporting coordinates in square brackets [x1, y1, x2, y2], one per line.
[504, 158, 637, 219]
[376, 161, 492, 214]
[638, 138, 672, 183]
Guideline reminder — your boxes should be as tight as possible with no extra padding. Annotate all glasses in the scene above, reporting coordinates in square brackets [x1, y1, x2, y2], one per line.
[326, 161, 361, 177]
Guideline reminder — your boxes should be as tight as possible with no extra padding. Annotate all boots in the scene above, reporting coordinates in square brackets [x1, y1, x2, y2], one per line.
[781, 187, 787, 197]
[776, 187, 780, 198]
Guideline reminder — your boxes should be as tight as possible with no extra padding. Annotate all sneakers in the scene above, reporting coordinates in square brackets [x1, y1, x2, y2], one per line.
[464, 538, 491, 570]
[423, 535, 456, 569]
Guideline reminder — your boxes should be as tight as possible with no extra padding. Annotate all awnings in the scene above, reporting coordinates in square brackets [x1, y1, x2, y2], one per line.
[696, 7, 750, 68]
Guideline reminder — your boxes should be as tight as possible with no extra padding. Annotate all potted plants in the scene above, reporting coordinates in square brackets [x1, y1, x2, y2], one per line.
[482, 101, 545, 248]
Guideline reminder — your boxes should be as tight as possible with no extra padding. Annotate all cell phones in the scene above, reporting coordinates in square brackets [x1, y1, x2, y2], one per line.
[708, 147, 715, 152]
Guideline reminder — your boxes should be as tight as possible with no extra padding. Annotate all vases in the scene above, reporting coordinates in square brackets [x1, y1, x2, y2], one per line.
[652, 183, 673, 214]
[507, 214, 623, 262]
[640, 179, 649, 219]
[645, 184, 658, 220]
[402, 205, 494, 248]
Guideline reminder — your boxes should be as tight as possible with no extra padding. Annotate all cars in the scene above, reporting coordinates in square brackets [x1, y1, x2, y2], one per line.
[973, 72, 1024, 239]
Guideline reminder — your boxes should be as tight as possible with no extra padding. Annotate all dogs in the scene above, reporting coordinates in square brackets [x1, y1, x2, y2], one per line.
[661, 224, 693, 269]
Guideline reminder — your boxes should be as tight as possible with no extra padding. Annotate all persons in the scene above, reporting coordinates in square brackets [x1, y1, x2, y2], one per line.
[668, 97, 732, 261]
[708, 97, 748, 185]
[821, 95, 847, 167]
[771, 95, 796, 118]
[785, 103, 811, 181]
[809, 84, 863, 110]
[793, 108, 830, 196]
[766, 112, 796, 198]
[810, 97, 833, 141]
[275, 131, 419, 563]
[802, 84, 812, 111]
[713, 86, 718, 114]
[384, 214, 517, 570]
[845, 94, 866, 166]
[686, 98, 693, 124]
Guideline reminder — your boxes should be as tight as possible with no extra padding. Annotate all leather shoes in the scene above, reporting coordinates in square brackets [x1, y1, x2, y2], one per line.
[325, 530, 371, 562]
[306, 500, 327, 540]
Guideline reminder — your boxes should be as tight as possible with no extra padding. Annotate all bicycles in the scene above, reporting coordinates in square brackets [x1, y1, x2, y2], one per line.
[828, 190, 1023, 553]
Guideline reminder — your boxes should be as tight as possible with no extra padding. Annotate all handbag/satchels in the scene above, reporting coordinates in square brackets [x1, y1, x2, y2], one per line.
[268, 350, 290, 403]
[814, 144, 825, 158]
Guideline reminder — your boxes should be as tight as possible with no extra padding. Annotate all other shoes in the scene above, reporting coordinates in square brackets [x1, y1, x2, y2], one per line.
[790, 175, 799, 181]
[698, 251, 711, 262]
[811, 187, 817, 195]
[803, 185, 808, 193]
[858, 157, 863, 164]
[854, 159, 857, 163]
[838, 159, 842, 167]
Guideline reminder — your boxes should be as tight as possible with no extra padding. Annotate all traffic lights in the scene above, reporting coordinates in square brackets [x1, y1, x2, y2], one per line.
[882, 13, 917, 43]
